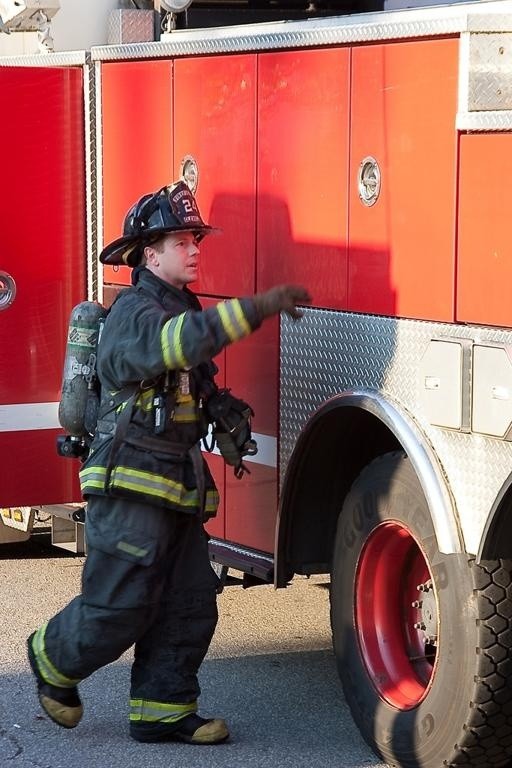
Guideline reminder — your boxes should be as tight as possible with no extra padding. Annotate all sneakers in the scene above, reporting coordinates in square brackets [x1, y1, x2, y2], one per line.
[130, 713, 230, 745]
[27, 630, 84, 729]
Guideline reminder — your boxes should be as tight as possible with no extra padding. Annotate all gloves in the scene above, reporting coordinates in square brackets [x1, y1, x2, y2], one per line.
[253, 283, 311, 320]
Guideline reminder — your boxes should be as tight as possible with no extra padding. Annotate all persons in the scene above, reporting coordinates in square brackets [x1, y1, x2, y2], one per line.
[29, 178, 314, 743]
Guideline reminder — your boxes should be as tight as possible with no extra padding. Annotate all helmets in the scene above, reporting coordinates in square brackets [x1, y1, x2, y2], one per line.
[98, 180, 224, 265]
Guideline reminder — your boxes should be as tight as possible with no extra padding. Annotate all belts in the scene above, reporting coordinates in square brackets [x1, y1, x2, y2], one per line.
[95, 419, 207, 521]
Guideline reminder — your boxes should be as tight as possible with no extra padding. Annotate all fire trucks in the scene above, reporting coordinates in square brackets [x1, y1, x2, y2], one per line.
[0, 0, 512, 768]
